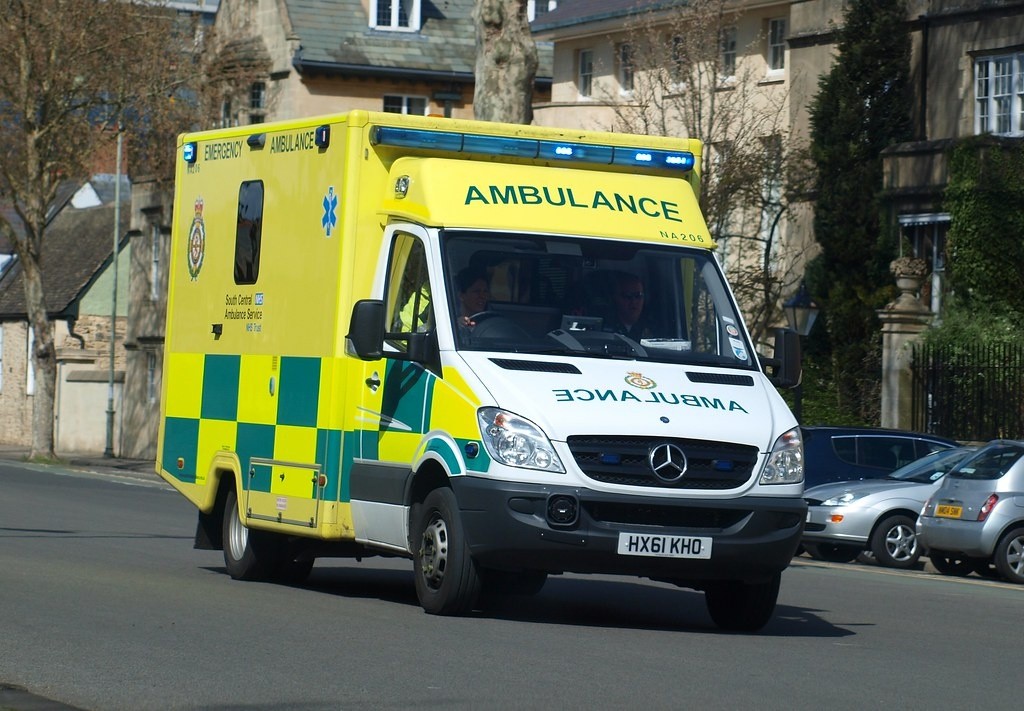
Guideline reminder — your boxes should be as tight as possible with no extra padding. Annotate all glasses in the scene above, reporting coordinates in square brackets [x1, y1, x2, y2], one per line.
[615, 289, 645, 302]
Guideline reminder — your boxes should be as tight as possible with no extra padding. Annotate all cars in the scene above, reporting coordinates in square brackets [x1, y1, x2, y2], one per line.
[913, 439, 1024, 585]
[803, 444, 984, 568]
[801, 426, 963, 493]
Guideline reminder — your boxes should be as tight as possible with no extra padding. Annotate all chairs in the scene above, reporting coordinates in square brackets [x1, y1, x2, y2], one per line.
[877, 450, 898, 469]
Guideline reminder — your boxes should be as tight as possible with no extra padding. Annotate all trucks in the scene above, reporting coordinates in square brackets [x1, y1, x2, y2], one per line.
[154, 113, 804, 629]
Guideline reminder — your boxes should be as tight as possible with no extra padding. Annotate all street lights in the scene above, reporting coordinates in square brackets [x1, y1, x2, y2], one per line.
[781, 278, 811, 425]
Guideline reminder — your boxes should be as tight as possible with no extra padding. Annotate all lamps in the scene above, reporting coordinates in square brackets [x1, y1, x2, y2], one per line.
[782, 279, 821, 337]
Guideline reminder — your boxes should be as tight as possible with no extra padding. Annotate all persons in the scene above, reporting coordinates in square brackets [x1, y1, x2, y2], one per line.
[595, 272, 668, 360]
[453, 268, 496, 347]
